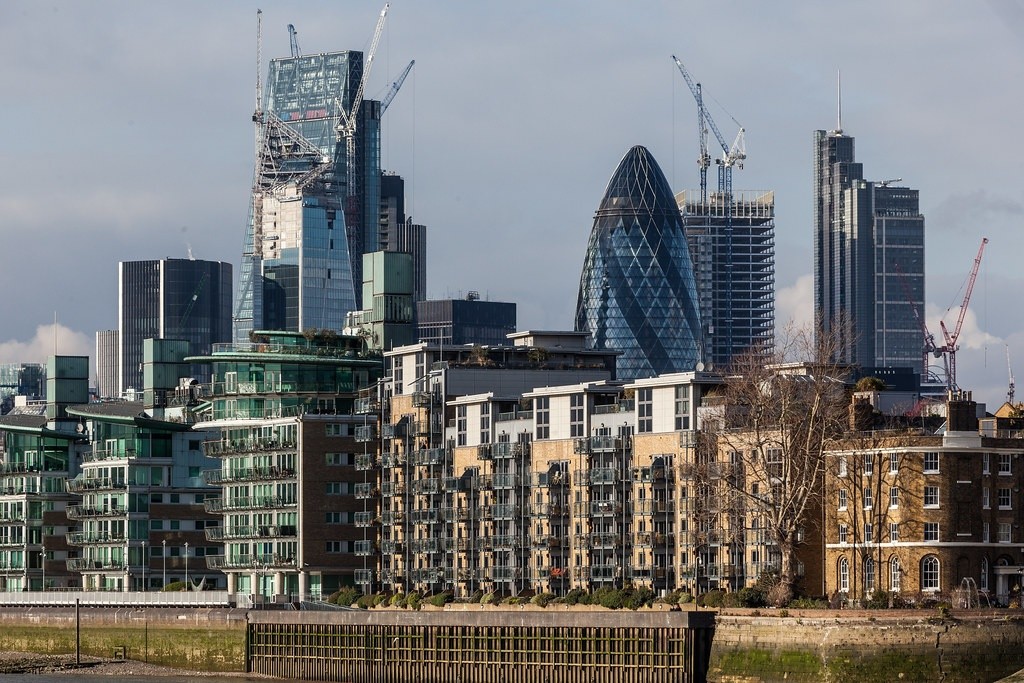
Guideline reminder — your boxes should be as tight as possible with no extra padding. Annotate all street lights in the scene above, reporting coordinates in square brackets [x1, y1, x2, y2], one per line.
[162, 539, 167, 592]
[695, 556, 702, 610]
[184, 542, 189, 591]
[39, 546, 47, 592]
[141, 541, 146, 591]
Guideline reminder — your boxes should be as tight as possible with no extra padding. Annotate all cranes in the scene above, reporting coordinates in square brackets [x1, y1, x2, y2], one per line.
[668, 52, 749, 211]
[1005, 343, 1016, 403]
[891, 236, 989, 402]
[242, 0, 416, 197]
